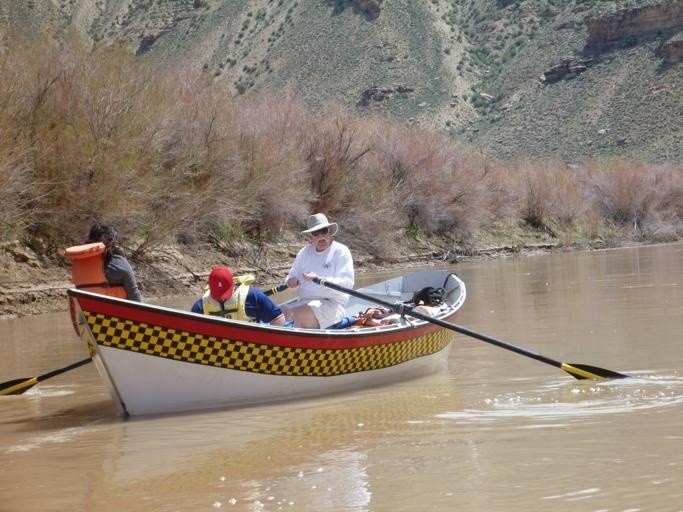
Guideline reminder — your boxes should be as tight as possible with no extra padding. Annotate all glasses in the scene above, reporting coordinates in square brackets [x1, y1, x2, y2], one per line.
[309, 227, 331, 238]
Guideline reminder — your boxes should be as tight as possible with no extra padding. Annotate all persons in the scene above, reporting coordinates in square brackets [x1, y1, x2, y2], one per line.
[86, 223, 141, 302]
[191, 267, 285, 326]
[280, 213, 354, 329]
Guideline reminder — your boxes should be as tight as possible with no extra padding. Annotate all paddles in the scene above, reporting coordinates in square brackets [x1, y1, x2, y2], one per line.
[301, 273, 630, 381]
[0, 284, 287, 395]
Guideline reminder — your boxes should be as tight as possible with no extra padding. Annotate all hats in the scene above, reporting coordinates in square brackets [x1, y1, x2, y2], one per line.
[208, 268, 233, 302]
[300, 212, 338, 240]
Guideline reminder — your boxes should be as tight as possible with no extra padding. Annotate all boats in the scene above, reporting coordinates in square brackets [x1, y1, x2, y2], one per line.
[67, 270, 466, 417]
